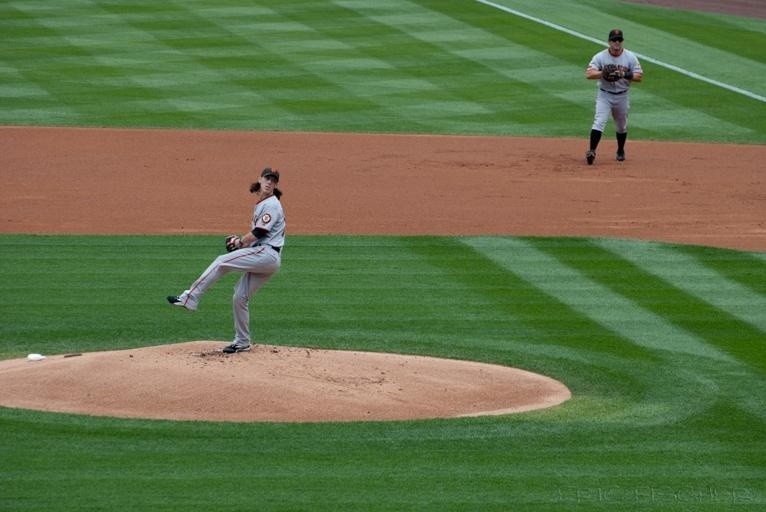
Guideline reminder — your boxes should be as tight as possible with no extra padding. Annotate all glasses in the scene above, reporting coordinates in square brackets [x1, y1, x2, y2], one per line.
[612, 38, 622, 42]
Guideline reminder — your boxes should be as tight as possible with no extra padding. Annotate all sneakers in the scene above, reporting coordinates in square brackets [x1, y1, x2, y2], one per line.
[166, 295, 198, 311]
[223, 342, 250, 353]
[586, 151, 595, 165]
[615, 150, 625, 161]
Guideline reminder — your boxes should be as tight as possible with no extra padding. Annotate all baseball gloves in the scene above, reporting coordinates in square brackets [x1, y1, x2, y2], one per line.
[226, 235, 240, 251]
[603, 64, 623, 83]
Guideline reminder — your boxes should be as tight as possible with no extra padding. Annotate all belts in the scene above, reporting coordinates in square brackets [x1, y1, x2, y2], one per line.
[252, 242, 280, 252]
[600, 88, 628, 95]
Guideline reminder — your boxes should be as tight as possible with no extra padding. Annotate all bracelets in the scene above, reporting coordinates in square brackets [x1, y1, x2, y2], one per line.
[234, 238, 244, 249]
[624, 71, 633, 80]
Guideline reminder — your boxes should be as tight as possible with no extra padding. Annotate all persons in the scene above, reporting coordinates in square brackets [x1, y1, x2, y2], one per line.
[585, 29, 643, 165]
[167, 167, 287, 353]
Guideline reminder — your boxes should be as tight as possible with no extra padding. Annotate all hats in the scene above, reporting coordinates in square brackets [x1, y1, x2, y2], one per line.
[261, 167, 279, 182]
[609, 29, 623, 41]
